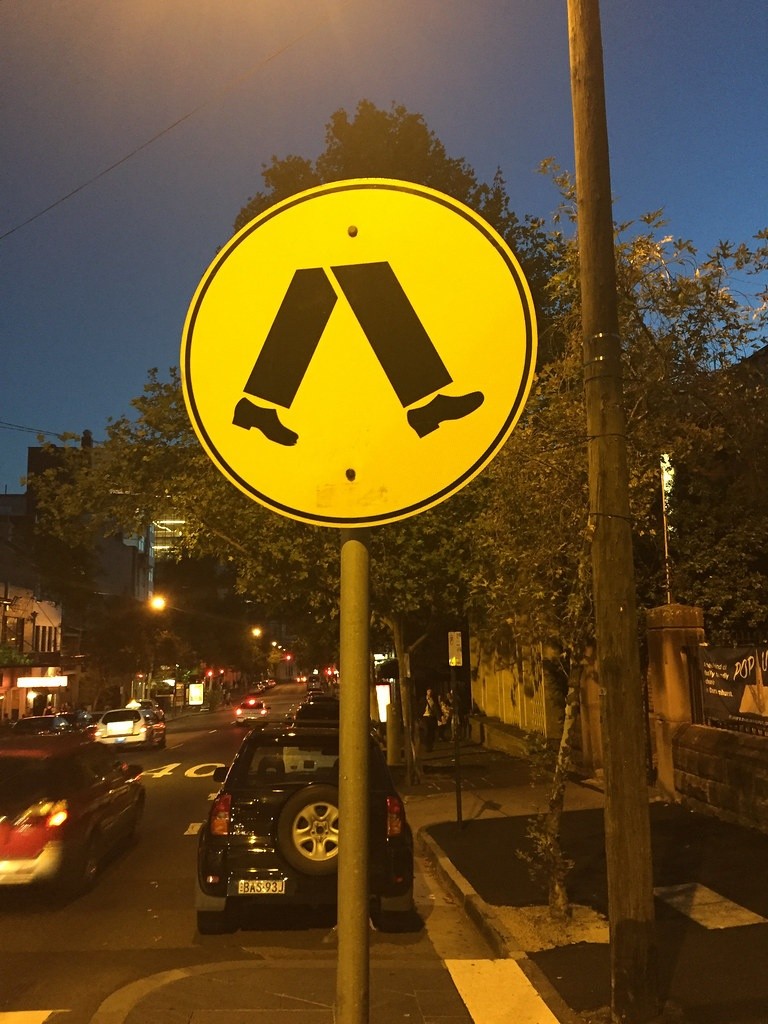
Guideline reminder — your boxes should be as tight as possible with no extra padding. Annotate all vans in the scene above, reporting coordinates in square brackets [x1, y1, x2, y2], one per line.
[307, 675, 323, 691]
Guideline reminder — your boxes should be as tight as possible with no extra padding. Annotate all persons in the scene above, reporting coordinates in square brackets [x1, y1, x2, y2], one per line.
[222, 686, 234, 709]
[417, 686, 444, 753]
[22, 707, 33, 718]
[61, 701, 71, 712]
[43, 702, 59, 716]
[2, 713, 15, 728]
[437, 692, 460, 742]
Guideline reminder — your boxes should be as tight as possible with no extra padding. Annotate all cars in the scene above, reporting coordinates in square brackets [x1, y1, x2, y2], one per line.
[234, 697, 271, 727]
[291, 689, 342, 720]
[246, 678, 277, 696]
[0, 735, 147, 904]
[294, 674, 308, 684]
[127, 698, 166, 722]
[92, 708, 168, 752]
[1, 708, 99, 741]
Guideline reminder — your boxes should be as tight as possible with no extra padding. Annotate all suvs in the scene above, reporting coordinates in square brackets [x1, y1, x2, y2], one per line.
[195, 719, 416, 935]
